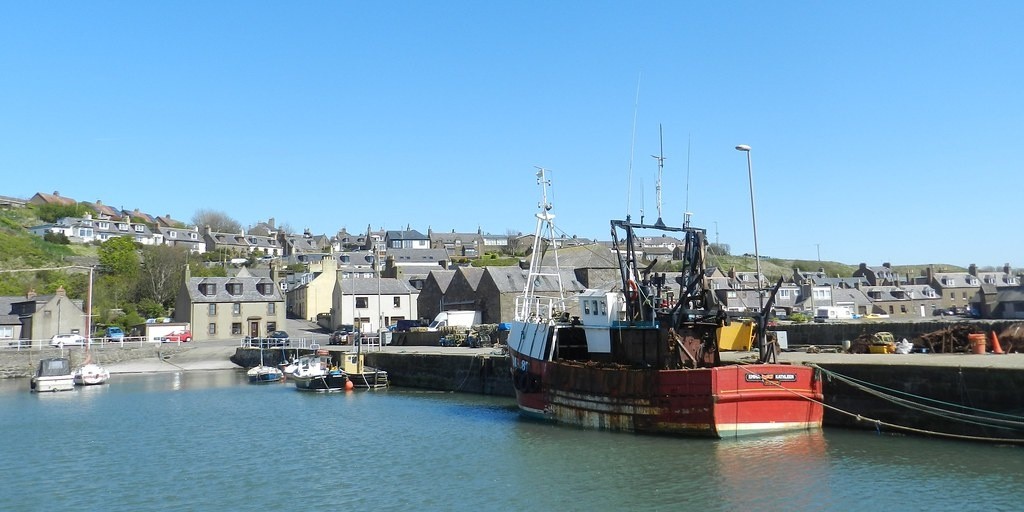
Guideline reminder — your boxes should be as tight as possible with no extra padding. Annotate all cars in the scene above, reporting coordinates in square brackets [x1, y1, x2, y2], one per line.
[397, 320, 428, 332]
[271, 331, 290, 346]
[163, 330, 192, 342]
[49, 334, 92, 348]
[329, 331, 349, 344]
[338, 325, 361, 335]
[933, 306, 972, 318]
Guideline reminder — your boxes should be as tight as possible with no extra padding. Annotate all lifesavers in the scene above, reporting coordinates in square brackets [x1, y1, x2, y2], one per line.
[513, 368, 534, 393]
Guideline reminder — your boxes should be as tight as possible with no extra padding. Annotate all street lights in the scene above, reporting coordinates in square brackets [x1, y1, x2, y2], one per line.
[736, 145, 768, 354]
[371, 234, 382, 351]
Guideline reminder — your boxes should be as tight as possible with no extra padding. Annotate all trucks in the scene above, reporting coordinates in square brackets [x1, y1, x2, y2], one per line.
[428, 311, 482, 332]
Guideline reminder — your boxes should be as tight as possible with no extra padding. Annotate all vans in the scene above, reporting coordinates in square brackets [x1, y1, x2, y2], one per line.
[106, 327, 124, 341]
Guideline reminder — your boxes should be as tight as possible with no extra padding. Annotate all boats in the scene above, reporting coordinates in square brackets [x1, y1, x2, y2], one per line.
[506, 123, 824, 439]
[283, 349, 333, 379]
[339, 312, 390, 388]
[292, 357, 345, 393]
[74, 363, 110, 384]
[30, 358, 75, 392]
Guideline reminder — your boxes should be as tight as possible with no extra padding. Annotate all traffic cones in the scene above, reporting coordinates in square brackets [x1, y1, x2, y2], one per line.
[992, 331, 1005, 354]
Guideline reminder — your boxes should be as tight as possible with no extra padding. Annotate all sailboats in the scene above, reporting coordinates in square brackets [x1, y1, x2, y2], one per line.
[246, 323, 282, 383]
